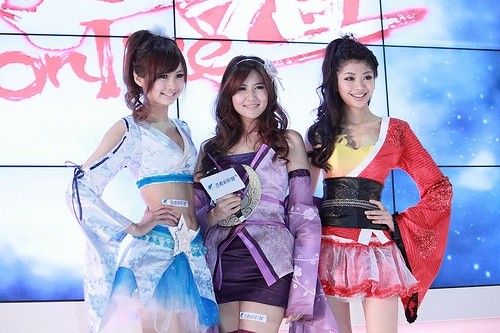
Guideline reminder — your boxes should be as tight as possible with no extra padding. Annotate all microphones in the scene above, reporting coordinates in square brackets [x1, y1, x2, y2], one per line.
[200, 157, 246, 223]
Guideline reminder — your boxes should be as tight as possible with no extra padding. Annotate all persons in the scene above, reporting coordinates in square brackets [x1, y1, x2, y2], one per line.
[305, 39, 453, 333]
[193, 55, 339, 333]
[65, 30, 222, 333]
